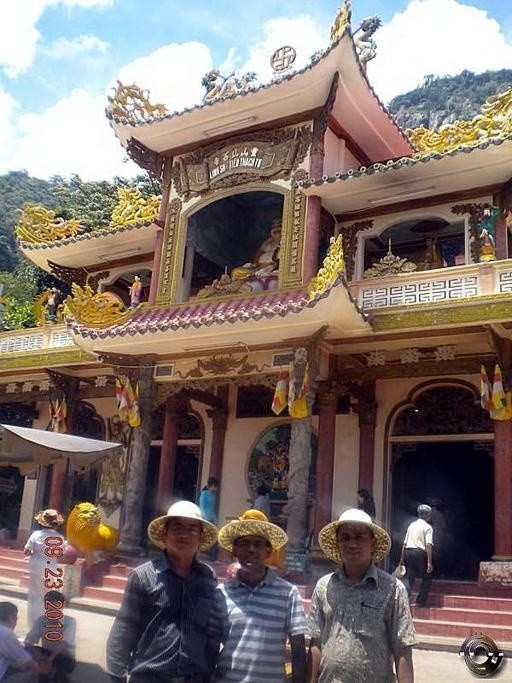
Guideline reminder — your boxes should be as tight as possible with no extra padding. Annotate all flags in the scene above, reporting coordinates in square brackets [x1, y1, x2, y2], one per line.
[270, 360, 313, 420]
[46, 393, 69, 433]
[115, 376, 142, 427]
[479, 362, 512, 421]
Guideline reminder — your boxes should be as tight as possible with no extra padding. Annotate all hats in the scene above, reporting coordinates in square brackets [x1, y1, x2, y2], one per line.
[216, 509, 289, 553]
[146, 499, 220, 552]
[35, 508, 65, 528]
[317, 508, 392, 564]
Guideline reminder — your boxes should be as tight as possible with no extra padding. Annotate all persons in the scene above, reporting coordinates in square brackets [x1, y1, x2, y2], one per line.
[411, 237, 443, 271]
[0, 600, 54, 683]
[48, 286, 60, 318]
[23, 507, 68, 635]
[249, 484, 272, 518]
[398, 503, 434, 608]
[355, 488, 377, 520]
[263, 438, 291, 492]
[105, 499, 220, 682]
[205, 509, 309, 683]
[425, 494, 448, 548]
[303, 506, 420, 682]
[102, 419, 127, 503]
[225, 552, 242, 580]
[198, 475, 219, 560]
[23, 591, 78, 683]
[228, 212, 283, 291]
[477, 206, 495, 261]
[128, 273, 142, 307]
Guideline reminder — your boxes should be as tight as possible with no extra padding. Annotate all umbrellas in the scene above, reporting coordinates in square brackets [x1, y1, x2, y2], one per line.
[0, 423, 124, 475]
